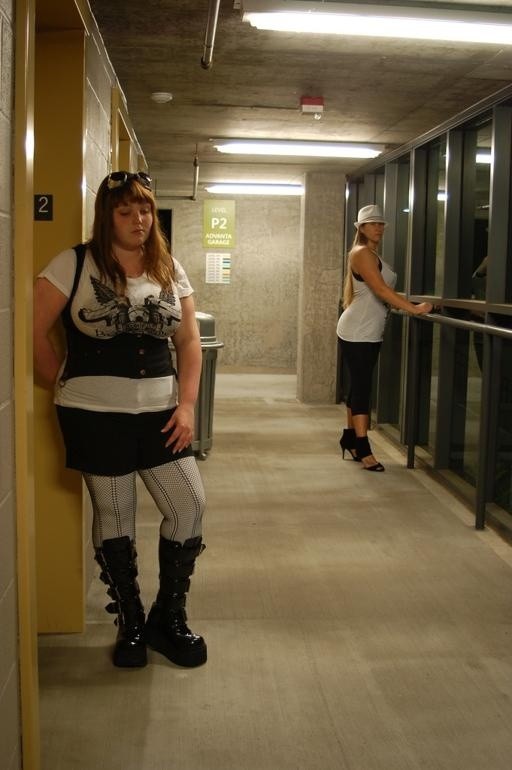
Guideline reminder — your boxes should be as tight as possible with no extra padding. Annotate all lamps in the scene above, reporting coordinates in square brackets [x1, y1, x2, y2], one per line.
[211, 138, 387, 160]
[231, 0, 512, 50]
[203, 182, 304, 197]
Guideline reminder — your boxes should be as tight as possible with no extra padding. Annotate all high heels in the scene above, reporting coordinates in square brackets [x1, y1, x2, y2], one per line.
[354, 435, 385, 472]
[339, 428, 361, 461]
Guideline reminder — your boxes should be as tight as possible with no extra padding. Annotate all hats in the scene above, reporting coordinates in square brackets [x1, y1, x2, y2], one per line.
[354, 204, 389, 229]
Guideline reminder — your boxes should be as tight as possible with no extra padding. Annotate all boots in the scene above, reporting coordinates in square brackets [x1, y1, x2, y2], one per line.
[93, 535, 148, 669]
[144, 533, 208, 668]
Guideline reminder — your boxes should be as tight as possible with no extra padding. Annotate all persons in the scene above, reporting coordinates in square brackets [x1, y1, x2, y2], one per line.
[33, 170, 210, 666]
[335, 204, 433, 472]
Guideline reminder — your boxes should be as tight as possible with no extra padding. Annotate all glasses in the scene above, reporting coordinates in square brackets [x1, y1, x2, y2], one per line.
[106, 171, 153, 191]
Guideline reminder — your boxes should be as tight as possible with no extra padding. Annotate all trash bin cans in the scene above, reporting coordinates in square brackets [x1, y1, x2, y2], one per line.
[168, 312, 224, 461]
